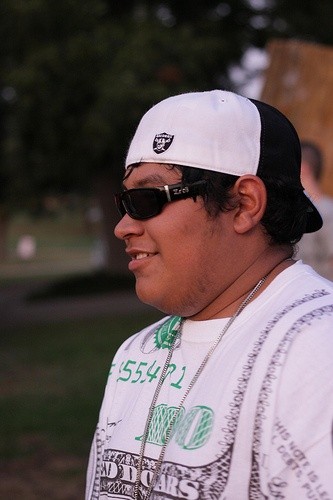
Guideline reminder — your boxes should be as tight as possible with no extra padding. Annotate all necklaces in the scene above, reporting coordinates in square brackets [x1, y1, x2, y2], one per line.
[134, 256, 293, 499]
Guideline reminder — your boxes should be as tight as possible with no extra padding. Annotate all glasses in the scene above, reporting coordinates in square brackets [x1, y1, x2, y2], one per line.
[112, 179, 236, 221]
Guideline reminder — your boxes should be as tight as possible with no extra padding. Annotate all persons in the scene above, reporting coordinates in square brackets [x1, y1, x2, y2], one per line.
[85, 90, 332, 500]
[295, 140, 332, 281]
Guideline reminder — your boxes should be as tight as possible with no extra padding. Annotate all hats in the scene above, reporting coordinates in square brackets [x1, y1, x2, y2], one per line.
[125, 89, 323, 234]
